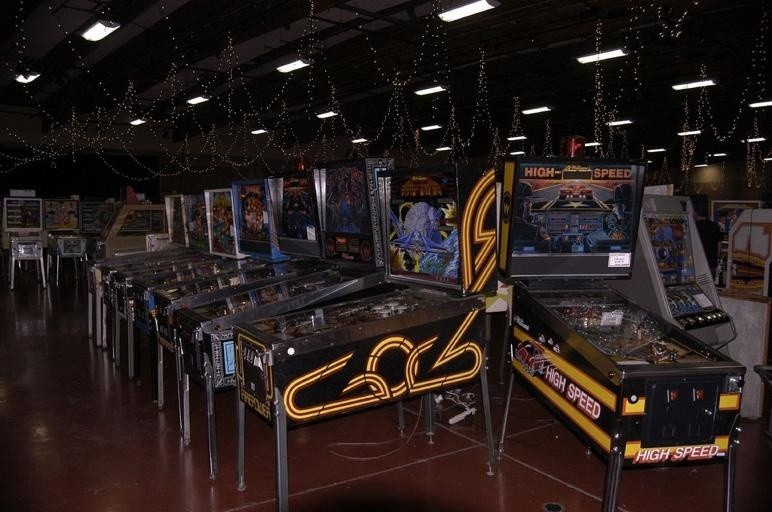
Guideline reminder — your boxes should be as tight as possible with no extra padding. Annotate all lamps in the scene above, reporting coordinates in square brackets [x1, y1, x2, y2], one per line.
[437, 0, 501, 23]
[13, 68, 40, 87]
[80, 18, 119, 47]
[130, 45, 772, 172]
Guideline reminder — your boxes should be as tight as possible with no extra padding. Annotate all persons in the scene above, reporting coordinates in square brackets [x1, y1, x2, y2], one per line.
[604, 183, 632, 240]
[688, 192, 725, 281]
[512, 181, 550, 249]
